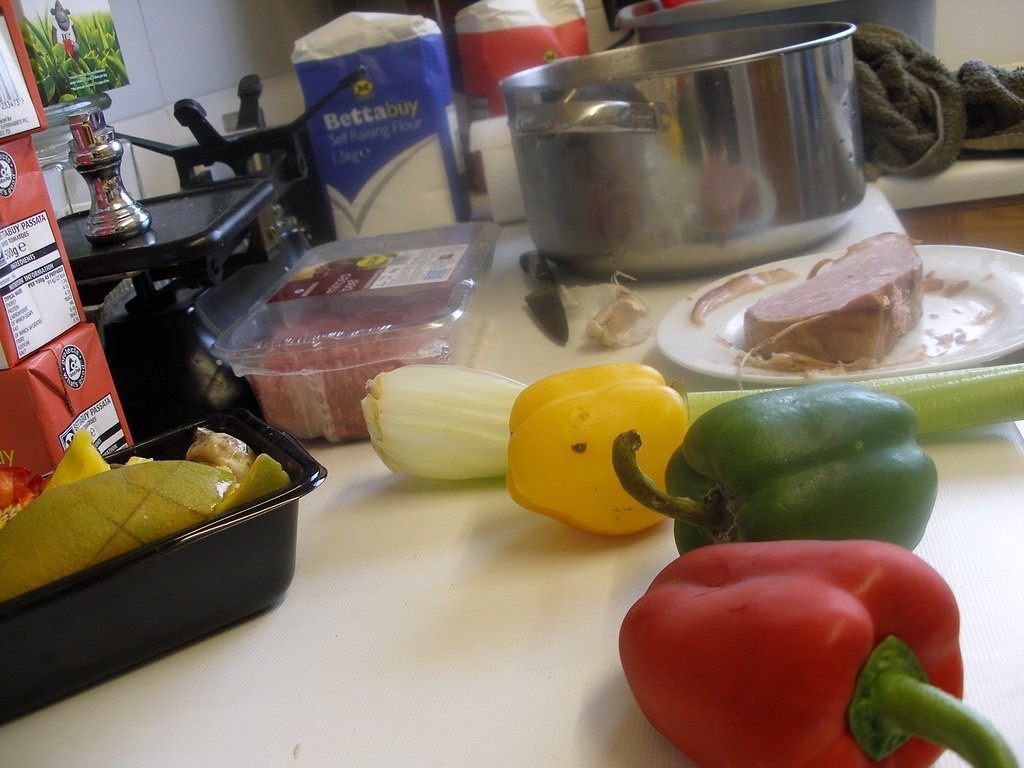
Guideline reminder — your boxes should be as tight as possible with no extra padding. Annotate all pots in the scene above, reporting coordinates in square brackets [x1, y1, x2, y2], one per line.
[498, 21, 866, 280]
[615, 0, 936, 58]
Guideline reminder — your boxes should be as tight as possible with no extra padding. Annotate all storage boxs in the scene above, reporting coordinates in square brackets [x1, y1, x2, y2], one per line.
[0, 0, 49, 145]
[0, 405, 328, 727]
[0, 321, 134, 477]
[0, 134, 88, 371]
[208, 220, 503, 444]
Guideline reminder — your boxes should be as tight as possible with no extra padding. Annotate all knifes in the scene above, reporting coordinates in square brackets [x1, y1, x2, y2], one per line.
[517, 249, 569, 346]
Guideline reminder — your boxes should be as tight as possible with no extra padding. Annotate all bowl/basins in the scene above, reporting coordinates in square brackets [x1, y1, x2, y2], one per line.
[0, 408, 328, 724]
[212, 221, 502, 444]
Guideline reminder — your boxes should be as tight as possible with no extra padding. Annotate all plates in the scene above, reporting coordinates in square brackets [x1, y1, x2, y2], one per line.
[656, 245, 1024, 385]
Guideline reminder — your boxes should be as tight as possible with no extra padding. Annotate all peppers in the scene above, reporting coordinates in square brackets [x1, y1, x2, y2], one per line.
[502, 364, 686, 535]
[617, 536, 1023, 768]
[612, 381, 939, 556]
